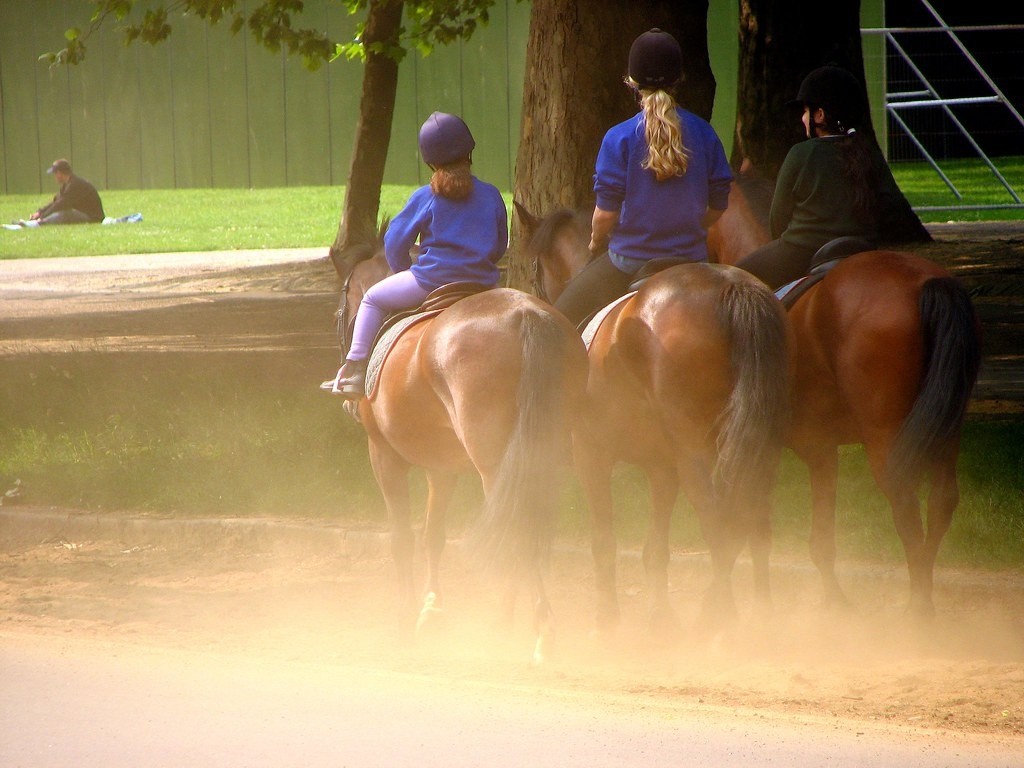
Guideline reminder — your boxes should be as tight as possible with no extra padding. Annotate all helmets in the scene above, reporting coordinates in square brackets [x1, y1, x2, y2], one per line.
[796, 67, 868, 128]
[419, 112, 475, 164]
[628, 28, 684, 88]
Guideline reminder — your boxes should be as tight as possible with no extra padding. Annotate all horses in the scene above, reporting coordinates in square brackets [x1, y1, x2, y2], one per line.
[328, 171, 978, 641]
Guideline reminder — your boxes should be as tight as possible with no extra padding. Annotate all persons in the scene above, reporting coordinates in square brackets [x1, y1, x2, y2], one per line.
[552, 27, 735, 327]
[332, 110, 508, 401]
[733, 67, 885, 291]
[2, 159, 105, 230]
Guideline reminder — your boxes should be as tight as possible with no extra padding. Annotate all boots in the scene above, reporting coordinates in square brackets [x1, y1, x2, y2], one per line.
[319, 360, 367, 397]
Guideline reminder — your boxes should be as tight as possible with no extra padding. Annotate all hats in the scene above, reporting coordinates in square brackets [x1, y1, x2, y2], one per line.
[47, 159, 70, 175]
